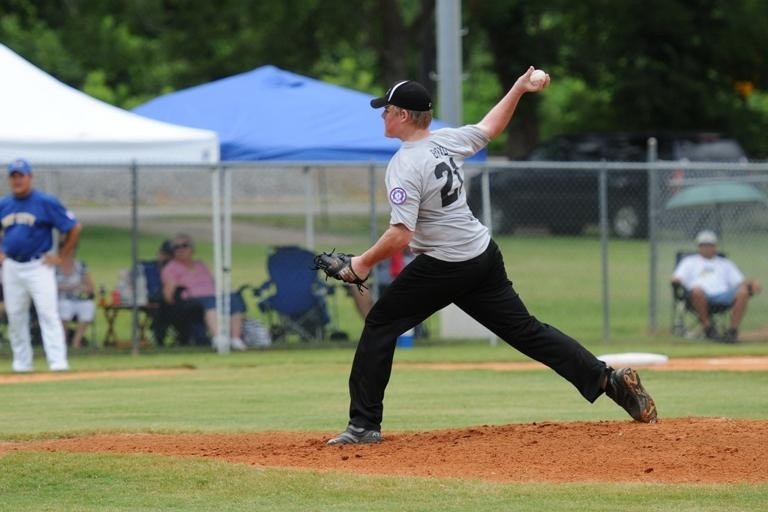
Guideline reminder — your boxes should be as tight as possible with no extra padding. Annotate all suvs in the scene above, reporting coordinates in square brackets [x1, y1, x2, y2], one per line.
[468, 127, 753, 239]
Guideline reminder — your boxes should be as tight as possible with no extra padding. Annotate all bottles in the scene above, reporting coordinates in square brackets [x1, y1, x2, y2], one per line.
[98, 285, 123, 305]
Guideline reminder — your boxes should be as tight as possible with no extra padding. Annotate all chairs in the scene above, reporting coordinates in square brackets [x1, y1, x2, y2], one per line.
[670, 250, 737, 343]
[60, 246, 332, 348]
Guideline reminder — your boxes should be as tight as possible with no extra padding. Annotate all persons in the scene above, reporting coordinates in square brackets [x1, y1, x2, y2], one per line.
[159, 233, 249, 351]
[321, 58, 657, 449]
[1, 157, 83, 372]
[351, 238, 433, 338]
[670, 228, 764, 343]
[55, 234, 97, 350]
[115, 239, 190, 349]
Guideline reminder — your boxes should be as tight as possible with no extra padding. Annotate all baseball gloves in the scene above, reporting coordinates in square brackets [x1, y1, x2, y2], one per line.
[313, 252, 372, 292]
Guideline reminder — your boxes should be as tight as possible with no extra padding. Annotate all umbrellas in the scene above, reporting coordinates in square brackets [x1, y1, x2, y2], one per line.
[664, 178, 767, 254]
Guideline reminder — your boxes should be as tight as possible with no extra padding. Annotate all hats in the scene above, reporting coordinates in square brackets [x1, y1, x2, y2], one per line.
[697, 230, 717, 246]
[370, 79, 432, 112]
[8, 159, 32, 176]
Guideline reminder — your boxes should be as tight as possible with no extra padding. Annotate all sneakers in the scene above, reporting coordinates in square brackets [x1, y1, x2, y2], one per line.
[327, 427, 383, 446]
[606, 368, 657, 423]
[705, 320, 740, 343]
[212, 335, 248, 351]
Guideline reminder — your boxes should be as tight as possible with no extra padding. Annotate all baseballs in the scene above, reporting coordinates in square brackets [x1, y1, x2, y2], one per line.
[529, 70, 546, 86]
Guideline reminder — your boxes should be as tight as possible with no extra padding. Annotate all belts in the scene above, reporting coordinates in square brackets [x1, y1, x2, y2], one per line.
[8, 254, 40, 262]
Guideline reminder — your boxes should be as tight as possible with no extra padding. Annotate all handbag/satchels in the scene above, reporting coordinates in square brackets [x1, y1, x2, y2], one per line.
[243, 318, 272, 348]
